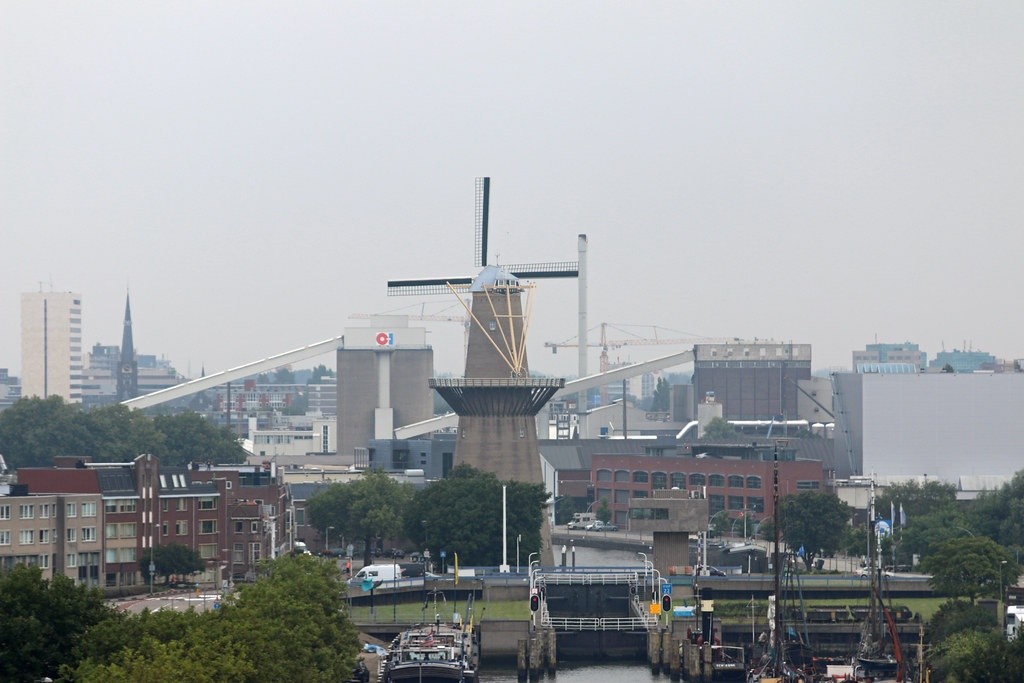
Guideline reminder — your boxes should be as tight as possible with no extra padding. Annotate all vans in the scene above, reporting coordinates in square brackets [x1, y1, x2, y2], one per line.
[567, 512, 597, 530]
[346, 564, 402, 586]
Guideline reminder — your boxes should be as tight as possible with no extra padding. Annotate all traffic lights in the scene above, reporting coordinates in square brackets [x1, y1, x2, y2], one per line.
[530, 594, 541, 612]
[661, 594, 673, 612]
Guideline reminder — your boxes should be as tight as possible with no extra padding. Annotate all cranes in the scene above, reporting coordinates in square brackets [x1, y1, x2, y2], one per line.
[545, 321, 773, 407]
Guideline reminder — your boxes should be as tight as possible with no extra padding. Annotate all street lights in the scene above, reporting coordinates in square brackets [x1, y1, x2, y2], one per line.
[223, 549, 231, 580]
[1000, 560, 1008, 602]
[325, 526, 335, 551]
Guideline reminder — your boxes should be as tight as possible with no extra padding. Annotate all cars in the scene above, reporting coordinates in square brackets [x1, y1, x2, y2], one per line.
[318, 546, 359, 561]
[370, 546, 405, 560]
[695, 565, 727, 577]
[585, 519, 619, 532]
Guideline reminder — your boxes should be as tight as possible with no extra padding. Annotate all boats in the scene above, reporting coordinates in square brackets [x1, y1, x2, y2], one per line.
[380, 584, 486, 683]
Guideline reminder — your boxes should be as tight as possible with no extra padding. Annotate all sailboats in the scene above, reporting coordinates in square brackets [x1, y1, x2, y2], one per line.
[679, 478, 938, 683]
